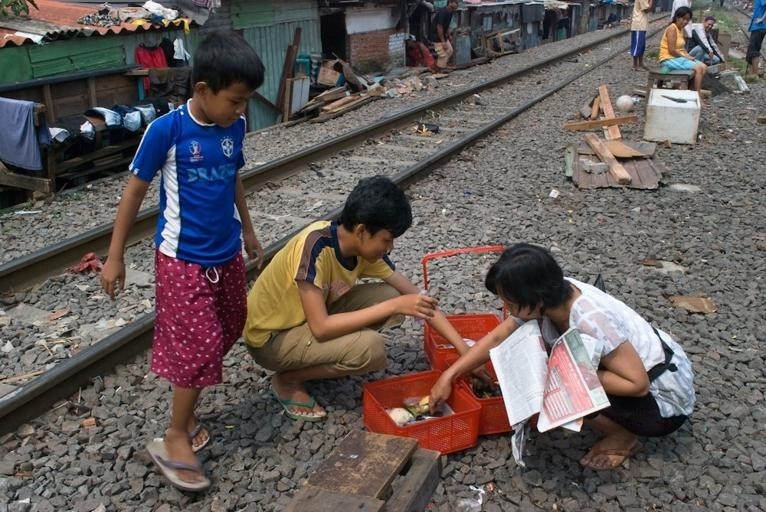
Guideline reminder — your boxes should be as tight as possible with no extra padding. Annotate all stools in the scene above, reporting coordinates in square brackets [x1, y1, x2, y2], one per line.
[643, 64, 695, 90]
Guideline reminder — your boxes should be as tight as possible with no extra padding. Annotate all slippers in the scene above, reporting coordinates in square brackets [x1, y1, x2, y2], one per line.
[271, 386, 327, 423]
[146, 422, 212, 493]
[580, 440, 645, 473]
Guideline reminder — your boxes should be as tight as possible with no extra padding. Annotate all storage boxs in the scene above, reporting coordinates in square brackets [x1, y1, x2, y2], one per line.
[642, 88, 703, 146]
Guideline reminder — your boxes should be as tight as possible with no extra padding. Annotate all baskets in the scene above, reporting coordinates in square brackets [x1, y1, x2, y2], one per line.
[362, 244, 521, 457]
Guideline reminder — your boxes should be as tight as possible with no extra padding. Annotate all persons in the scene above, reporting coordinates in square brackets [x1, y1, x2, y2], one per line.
[242, 174, 496, 421]
[688, 16, 726, 65]
[659, 6, 712, 110]
[630, 0, 653, 72]
[427, 242, 695, 469]
[741, 0, 766, 80]
[98, 27, 268, 494]
[429, 0, 458, 74]
[670, 0, 692, 52]
